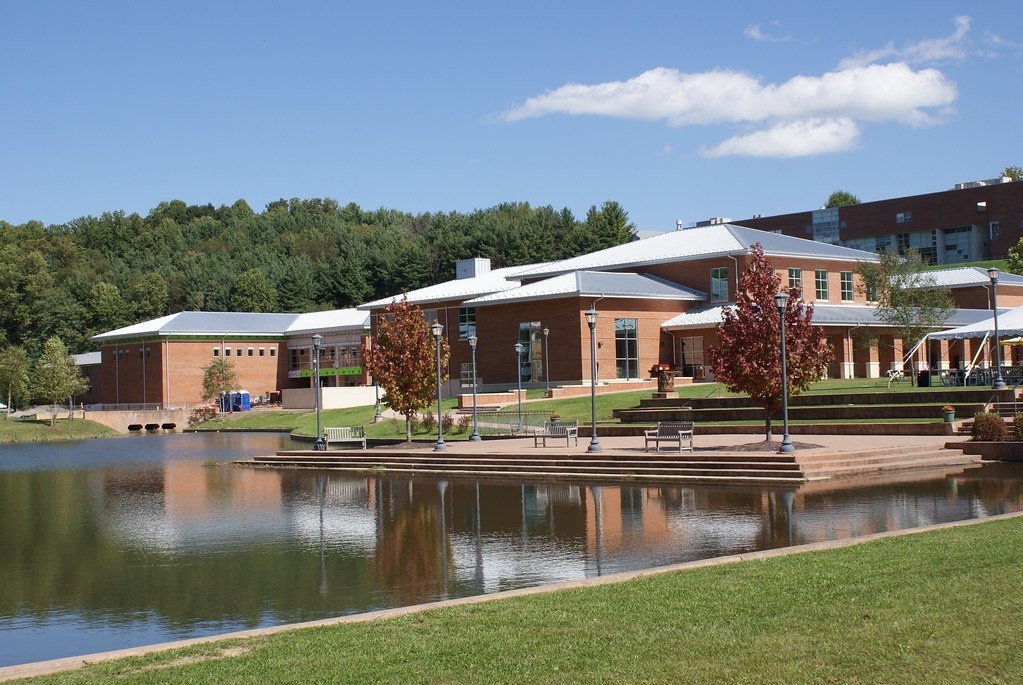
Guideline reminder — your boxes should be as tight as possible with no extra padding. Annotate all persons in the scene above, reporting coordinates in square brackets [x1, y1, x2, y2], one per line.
[1010, 362, 1022, 384]
[996, 361, 1009, 382]
[950, 356, 963, 386]
[973, 363, 984, 382]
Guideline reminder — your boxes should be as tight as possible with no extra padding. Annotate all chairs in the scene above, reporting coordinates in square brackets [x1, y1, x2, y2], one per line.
[937, 369, 990, 387]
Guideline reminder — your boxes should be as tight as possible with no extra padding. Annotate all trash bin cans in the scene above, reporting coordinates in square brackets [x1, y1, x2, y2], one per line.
[220, 389, 251, 412]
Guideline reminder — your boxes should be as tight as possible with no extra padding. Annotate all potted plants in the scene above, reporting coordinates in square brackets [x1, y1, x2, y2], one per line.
[940, 405, 955, 423]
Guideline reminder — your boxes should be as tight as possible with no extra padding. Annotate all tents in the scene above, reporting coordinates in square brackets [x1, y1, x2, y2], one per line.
[887, 306, 1023, 381]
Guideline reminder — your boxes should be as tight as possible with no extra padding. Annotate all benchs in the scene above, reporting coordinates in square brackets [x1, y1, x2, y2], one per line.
[533, 420, 578, 448]
[323, 425, 367, 450]
[643, 422, 694, 453]
[990, 366, 1023, 389]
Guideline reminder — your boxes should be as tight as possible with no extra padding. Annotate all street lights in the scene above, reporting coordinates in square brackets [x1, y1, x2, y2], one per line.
[584, 304, 602, 454]
[312, 333, 325, 451]
[513, 340, 524, 433]
[986, 265, 1009, 390]
[542, 325, 549, 398]
[430, 321, 448, 452]
[468, 334, 482, 441]
[773, 290, 796, 454]
[314, 472, 330, 598]
